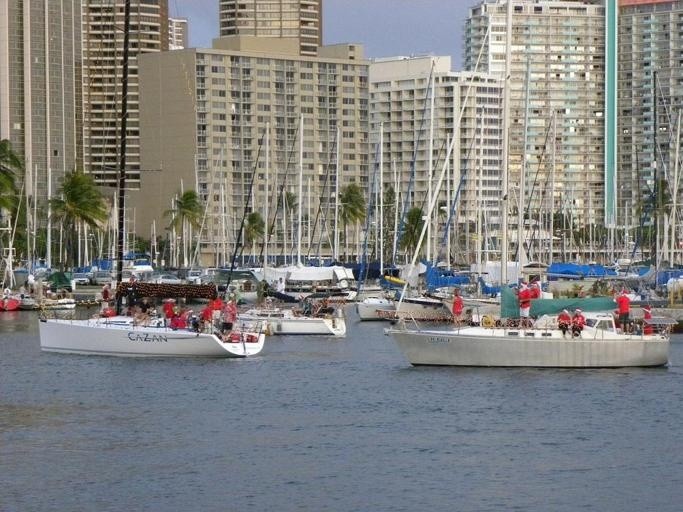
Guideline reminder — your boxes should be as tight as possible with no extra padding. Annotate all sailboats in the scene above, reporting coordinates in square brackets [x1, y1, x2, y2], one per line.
[258, 114, 357, 302]
[354, 121, 395, 319]
[388, 57, 492, 322]
[34, 0, 264, 359]
[242, 168, 292, 289]
[236, 122, 346, 338]
[186, 143, 226, 282]
[0, 214, 22, 311]
[353, 132, 460, 322]
[17, 163, 38, 309]
[221, 124, 350, 338]
[440, 55, 540, 321]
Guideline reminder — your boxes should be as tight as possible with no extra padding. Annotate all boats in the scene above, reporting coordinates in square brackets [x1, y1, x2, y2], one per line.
[38, 216, 76, 309]
[0, 282, 36, 310]
[28, 269, 76, 312]
[38, 303, 269, 357]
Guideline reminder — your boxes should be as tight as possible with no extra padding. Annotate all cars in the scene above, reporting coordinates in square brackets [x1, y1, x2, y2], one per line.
[71, 271, 92, 285]
[92, 270, 115, 286]
[186, 269, 203, 282]
[146, 273, 183, 287]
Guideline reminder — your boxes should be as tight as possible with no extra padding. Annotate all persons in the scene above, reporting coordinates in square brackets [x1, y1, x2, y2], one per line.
[443, 289, 464, 327]
[530, 282, 540, 299]
[291, 295, 330, 319]
[384, 290, 389, 301]
[394, 288, 402, 301]
[572, 307, 585, 336]
[274, 277, 286, 305]
[98, 281, 237, 336]
[642, 305, 653, 334]
[556, 309, 571, 338]
[517, 282, 529, 330]
[613, 291, 630, 335]
[263, 279, 269, 307]
[1, 272, 36, 310]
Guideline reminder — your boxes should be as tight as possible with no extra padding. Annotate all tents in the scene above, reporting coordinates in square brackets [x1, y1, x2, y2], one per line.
[223, 265, 355, 285]
